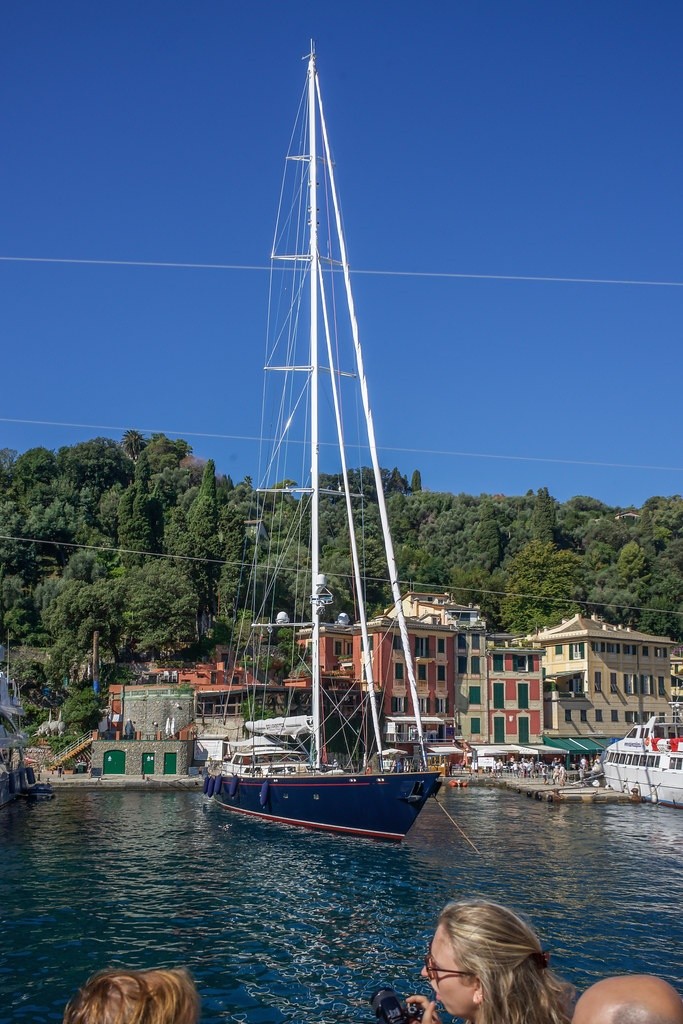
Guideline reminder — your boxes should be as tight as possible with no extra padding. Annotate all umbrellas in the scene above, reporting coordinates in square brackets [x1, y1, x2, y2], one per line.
[377, 748, 408, 755]
[166, 718, 170, 738]
[170, 718, 175, 738]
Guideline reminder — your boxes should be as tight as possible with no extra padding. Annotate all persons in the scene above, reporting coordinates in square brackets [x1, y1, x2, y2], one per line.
[448, 759, 466, 776]
[124, 720, 135, 734]
[61, 969, 199, 1024]
[405, 901, 577, 1024]
[334, 759, 337, 765]
[570, 974, 683, 1024]
[490, 755, 601, 785]
[59, 763, 62, 773]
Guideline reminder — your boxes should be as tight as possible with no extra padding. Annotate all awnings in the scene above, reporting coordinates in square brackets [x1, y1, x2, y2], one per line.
[512, 737, 612, 769]
[429, 747, 461, 752]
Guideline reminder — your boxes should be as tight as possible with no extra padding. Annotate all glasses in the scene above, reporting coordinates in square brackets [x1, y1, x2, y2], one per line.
[424, 941, 477, 983]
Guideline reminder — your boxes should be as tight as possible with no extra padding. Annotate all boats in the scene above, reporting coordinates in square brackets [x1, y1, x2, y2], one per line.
[449, 780, 467, 787]
[29, 784, 53, 801]
[0, 645, 36, 808]
[601, 678, 683, 809]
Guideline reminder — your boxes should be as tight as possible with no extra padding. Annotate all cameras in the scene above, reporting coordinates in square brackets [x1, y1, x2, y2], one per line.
[370, 987, 423, 1024]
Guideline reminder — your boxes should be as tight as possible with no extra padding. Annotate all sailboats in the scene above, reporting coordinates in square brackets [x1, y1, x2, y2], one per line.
[195, 31, 442, 845]
